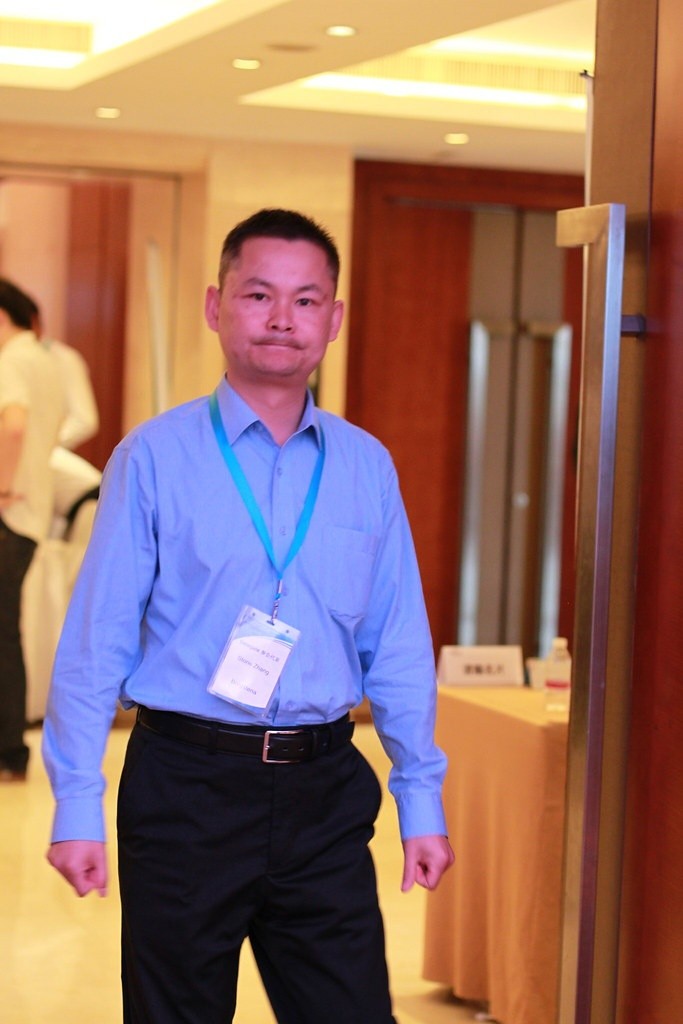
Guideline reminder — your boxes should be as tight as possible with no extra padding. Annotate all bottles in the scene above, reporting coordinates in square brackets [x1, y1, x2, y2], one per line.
[545, 637, 571, 711]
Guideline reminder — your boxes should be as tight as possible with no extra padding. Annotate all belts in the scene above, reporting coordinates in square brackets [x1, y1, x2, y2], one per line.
[137, 706, 356, 763]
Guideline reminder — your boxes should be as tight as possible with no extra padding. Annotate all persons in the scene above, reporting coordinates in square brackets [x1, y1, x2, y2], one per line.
[0, 276, 99, 783]
[42, 207, 456, 1024]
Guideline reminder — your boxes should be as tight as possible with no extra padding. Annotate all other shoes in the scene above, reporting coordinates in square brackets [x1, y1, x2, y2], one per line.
[0, 746, 30, 777]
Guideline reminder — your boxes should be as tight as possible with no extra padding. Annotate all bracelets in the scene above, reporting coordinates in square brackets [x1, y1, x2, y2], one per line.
[1, 490, 14, 498]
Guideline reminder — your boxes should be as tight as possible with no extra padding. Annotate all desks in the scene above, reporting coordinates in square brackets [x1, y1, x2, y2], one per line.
[421, 684, 571, 1024]
